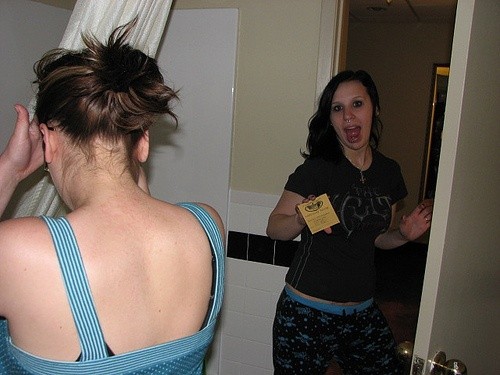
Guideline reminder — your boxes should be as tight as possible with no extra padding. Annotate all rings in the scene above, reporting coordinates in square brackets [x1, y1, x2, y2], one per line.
[425, 217, 428, 223]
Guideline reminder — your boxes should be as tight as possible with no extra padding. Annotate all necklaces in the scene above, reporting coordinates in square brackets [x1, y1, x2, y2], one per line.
[343, 147, 371, 184]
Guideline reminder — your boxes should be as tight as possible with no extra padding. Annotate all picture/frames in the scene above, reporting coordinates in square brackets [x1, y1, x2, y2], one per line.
[417, 63, 450, 205]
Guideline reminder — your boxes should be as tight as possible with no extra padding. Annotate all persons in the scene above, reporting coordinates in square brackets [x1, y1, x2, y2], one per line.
[266, 69, 434, 375]
[0, 13, 226, 375]
